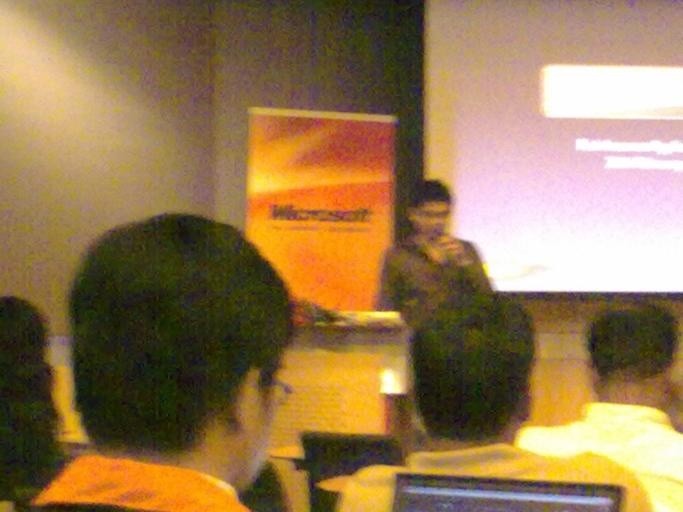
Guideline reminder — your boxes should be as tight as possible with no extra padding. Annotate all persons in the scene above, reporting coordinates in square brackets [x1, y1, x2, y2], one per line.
[0, 295, 70, 504]
[0, 212, 296, 511]
[336, 290, 655, 512]
[369, 179, 495, 331]
[512, 303, 683, 512]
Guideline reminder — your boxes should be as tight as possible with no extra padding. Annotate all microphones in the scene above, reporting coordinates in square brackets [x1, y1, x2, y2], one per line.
[432, 227, 464, 274]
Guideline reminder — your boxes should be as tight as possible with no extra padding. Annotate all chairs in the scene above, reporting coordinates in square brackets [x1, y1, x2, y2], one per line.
[299, 430, 405, 512]
[239, 460, 293, 512]
